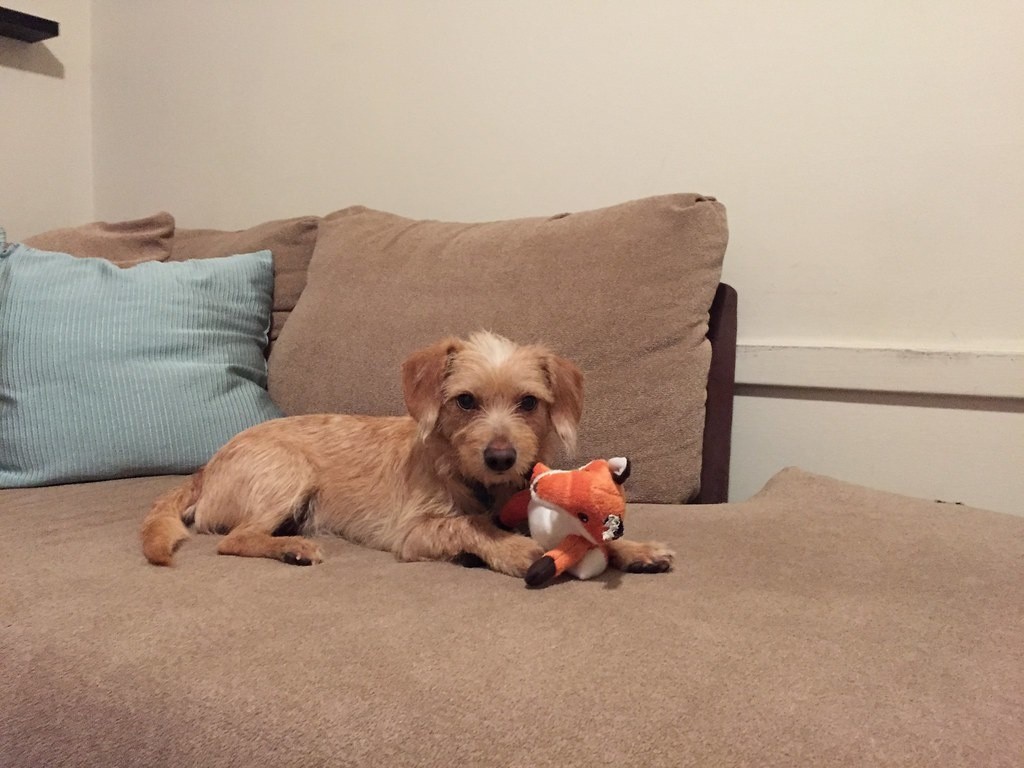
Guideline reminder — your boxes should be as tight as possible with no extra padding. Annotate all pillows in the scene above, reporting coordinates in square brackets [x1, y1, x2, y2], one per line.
[0, 193, 729, 504]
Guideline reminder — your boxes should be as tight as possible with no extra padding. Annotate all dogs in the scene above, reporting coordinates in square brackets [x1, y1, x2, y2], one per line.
[139, 330, 678, 578]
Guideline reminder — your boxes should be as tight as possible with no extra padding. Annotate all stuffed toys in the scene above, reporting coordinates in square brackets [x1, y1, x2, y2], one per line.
[501, 456, 630, 587]
